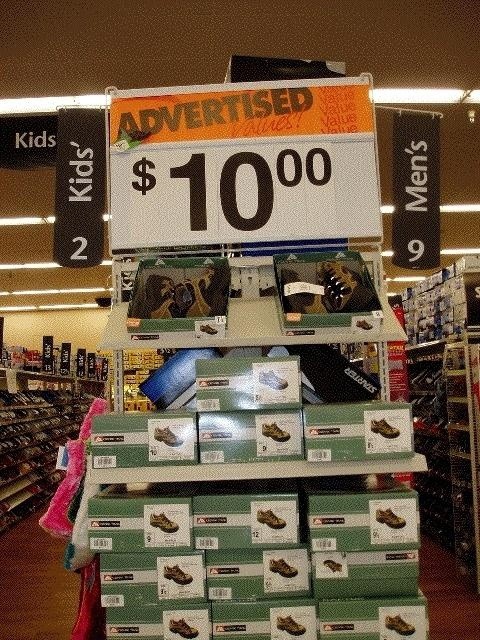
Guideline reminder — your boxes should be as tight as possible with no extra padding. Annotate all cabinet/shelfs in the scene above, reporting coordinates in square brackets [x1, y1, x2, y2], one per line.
[0, 365, 123, 536]
[347, 322, 480, 603]
[91, 71, 429, 638]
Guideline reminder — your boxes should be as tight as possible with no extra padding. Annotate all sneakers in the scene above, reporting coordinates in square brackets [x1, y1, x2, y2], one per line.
[150, 512, 180, 534]
[164, 563, 194, 585]
[153, 427, 183, 448]
[322, 559, 342, 573]
[375, 507, 407, 530]
[268, 557, 298, 579]
[276, 614, 307, 637]
[174, 276, 213, 317]
[385, 613, 416, 637]
[259, 370, 288, 391]
[261, 421, 292, 443]
[316, 260, 376, 312]
[256, 509, 287, 530]
[370, 417, 401, 440]
[281, 267, 327, 314]
[168, 617, 199, 639]
[140, 275, 177, 320]
